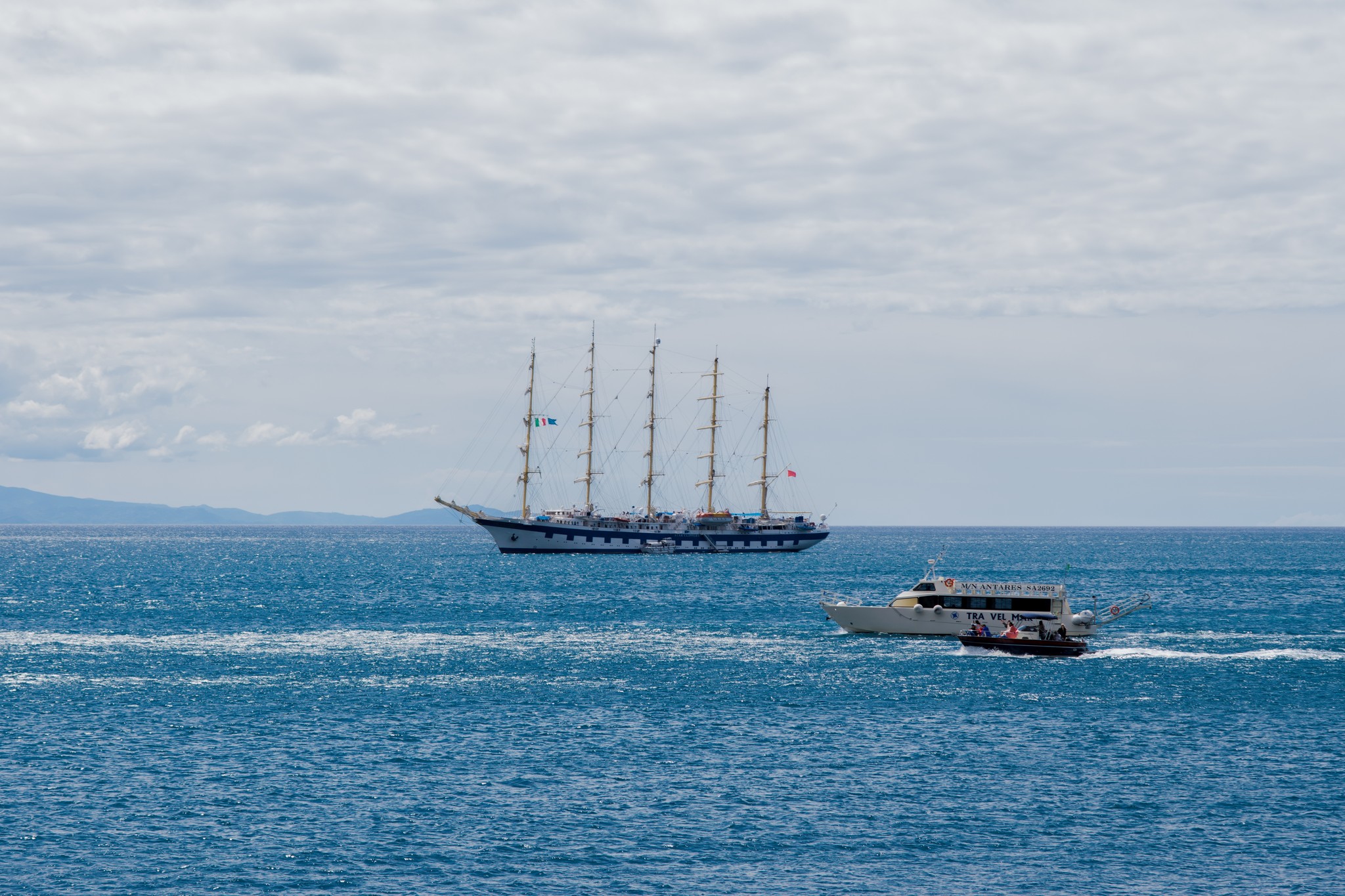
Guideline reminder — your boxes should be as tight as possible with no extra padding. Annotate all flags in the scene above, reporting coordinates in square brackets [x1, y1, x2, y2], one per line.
[1067, 564, 1070, 570]
[535, 417, 558, 428]
[787, 470, 796, 477]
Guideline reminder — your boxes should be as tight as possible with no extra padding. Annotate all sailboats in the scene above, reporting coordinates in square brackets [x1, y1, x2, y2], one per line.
[435, 321, 831, 555]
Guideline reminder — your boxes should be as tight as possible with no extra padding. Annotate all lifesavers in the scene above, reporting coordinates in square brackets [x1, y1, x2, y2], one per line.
[1110, 605, 1120, 615]
[945, 578, 954, 587]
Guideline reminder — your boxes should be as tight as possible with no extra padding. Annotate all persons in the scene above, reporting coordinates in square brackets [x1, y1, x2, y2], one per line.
[782, 516, 784, 518]
[1032, 620, 1067, 641]
[667, 543, 670, 546]
[1000, 619, 1017, 636]
[742, 512, 745, 518]
[967, 620, 991, 637]
[672, 543, 674, 546]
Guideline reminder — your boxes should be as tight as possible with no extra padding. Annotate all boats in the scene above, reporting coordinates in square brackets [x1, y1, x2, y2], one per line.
[958, 613, 1087, 658]
[818, 544, 1151, 636]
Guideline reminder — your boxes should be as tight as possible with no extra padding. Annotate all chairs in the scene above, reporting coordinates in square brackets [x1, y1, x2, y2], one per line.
[1005, 629, 1019, 638]
